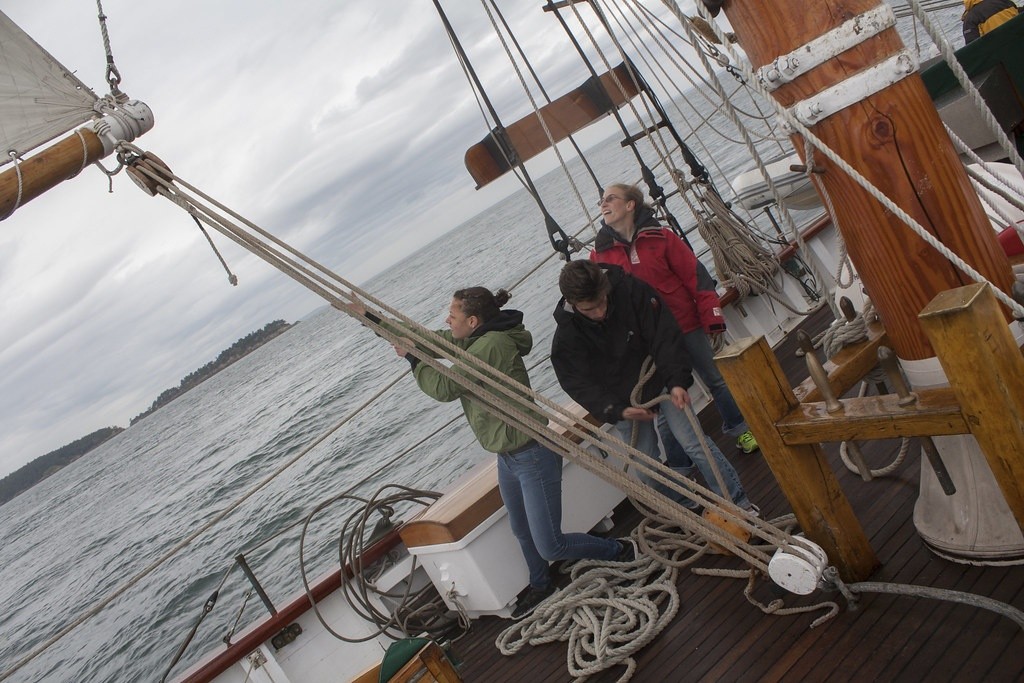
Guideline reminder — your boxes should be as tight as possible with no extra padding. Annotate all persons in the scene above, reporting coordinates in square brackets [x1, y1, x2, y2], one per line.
[332, 286, 638, 621]
[962, 0, 1019, 44]
[550, 259, 765, 530]
[589, 183, 758, 453]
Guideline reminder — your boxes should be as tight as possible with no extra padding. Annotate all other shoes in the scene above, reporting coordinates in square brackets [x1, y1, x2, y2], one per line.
[745, 509, 766, 528]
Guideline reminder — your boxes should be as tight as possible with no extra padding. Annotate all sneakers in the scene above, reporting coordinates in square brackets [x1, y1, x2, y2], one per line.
[615, 538, 638, 587]
[735, 430, 758, 453]
[511, 584, 560, 621]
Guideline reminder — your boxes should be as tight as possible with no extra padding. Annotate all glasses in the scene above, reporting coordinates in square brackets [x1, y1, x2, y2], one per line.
[597, 195, 625, 207]
